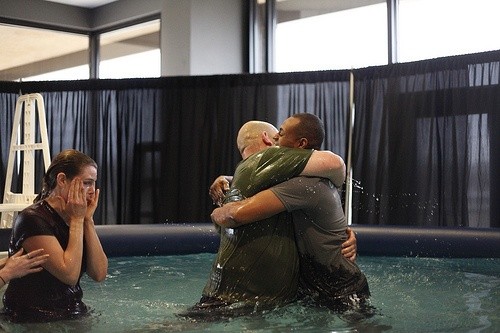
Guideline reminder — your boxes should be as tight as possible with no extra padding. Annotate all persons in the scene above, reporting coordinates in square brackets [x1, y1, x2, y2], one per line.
[209, 113, 371, 304]
[199, 121, 357, 305]
[0, 248, 49, 289]
[3, 150, 108, 323]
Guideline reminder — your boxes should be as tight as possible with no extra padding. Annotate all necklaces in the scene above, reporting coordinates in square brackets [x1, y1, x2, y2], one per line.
[43, 201, 70, 227]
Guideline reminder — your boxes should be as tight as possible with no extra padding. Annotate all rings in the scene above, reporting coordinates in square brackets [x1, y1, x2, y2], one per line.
[27, 253, 31, 259]
[218, 191, 223, 195]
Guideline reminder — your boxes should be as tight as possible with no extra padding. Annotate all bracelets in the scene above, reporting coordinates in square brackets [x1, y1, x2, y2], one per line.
[0, 276, 6, 285]
